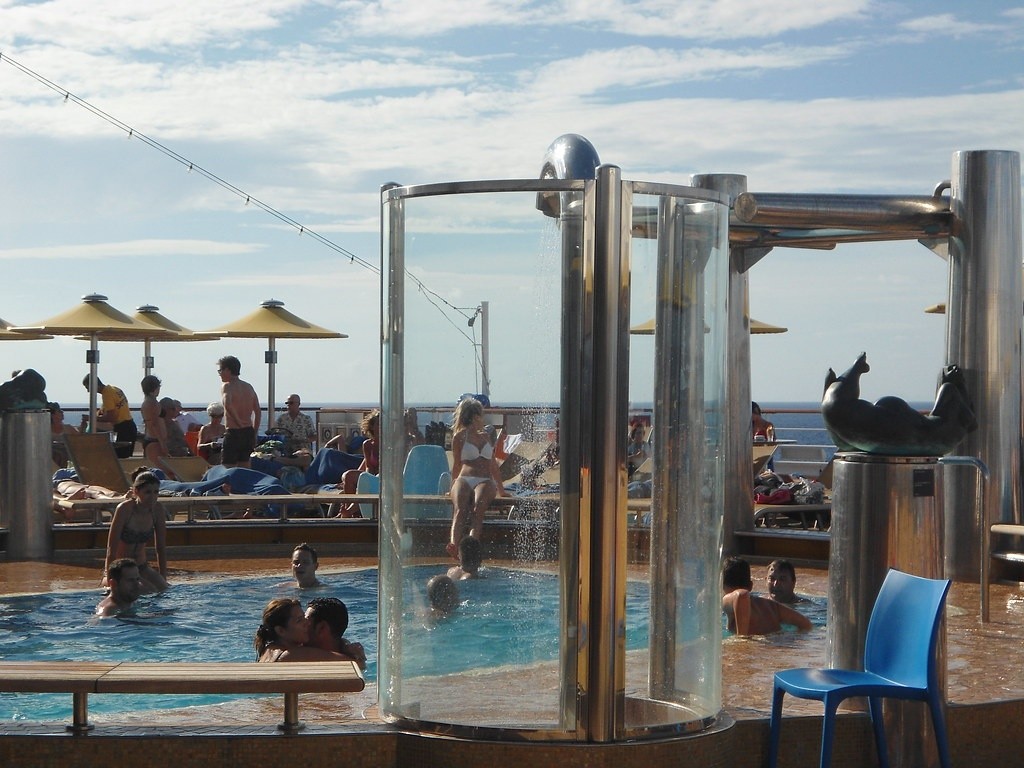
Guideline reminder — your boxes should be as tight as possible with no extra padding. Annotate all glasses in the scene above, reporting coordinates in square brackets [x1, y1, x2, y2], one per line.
[285, 401, 300, 406]
[218, 366, 227, 374]
[209, 413, 223, 418]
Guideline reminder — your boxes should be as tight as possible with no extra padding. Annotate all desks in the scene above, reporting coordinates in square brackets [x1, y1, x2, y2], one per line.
[54, 440, 134, 524]
[750, 439, 798, 471]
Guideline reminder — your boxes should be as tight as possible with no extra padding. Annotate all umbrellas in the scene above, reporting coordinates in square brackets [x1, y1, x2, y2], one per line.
[924, 304, 945, 314]
[0, 318, 53, 340]
[193, 298, 349, 429]
[629, 318, 788, 335]
[71, 306, 220, 376]
[7, 292, 178, 431]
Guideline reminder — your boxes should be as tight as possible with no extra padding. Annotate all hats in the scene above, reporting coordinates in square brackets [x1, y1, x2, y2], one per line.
[160, 398, 178, 409]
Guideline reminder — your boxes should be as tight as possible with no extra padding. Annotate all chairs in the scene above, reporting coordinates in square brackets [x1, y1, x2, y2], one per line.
[60, 431, 828, 527]
[767, 568, 951, 767]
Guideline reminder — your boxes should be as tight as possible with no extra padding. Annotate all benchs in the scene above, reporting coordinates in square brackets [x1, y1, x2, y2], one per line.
[1, 662, 362, 729]
[61, 494, 562, 520]
[626, 497, 651, 528]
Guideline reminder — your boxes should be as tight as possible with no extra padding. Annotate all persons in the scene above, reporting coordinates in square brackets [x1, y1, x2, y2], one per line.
[752, 401, 776, 437]
[97, 558, 141, 615]
[281, 544, 320, 587]
[759, 560, 810, 602]
[447, 399, 558, 561]
[627, 424, 651, 477]
[254, 598, 367, 670]
[427, 575, 459, 615]
[722, 558, 812, 635]
[100, 471, 170, 593]
[447, 537, 482, 580]
[51, 356, 424, 518]
